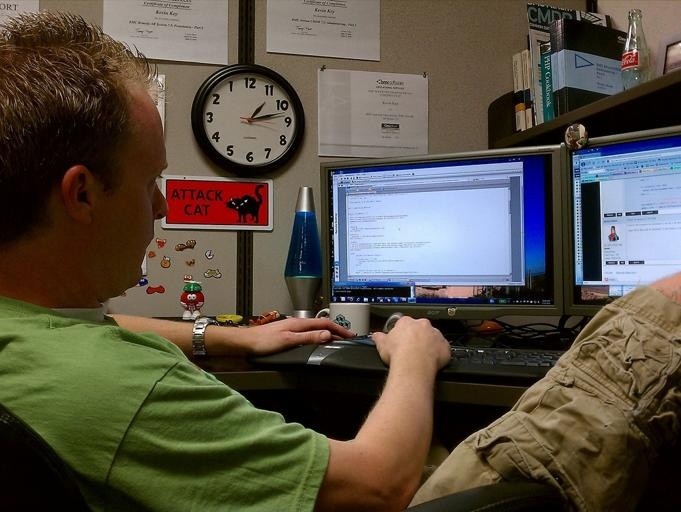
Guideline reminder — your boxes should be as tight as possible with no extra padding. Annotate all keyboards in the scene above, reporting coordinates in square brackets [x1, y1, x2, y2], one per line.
[438, 347, 564, 381]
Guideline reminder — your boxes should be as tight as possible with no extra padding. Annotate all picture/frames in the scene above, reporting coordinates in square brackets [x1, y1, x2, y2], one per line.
[162, 175, 276, 233]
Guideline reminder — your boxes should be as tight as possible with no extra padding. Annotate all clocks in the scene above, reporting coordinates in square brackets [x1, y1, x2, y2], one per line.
[188, 58, 307, 181]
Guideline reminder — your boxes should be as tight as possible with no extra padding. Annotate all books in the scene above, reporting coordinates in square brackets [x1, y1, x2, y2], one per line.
[525, 2, 612, 125]
[540, 39, 553, 121]
[520, 49, 535, 130]
[511, 52, 523, 130]
[551, 18, 635, 117]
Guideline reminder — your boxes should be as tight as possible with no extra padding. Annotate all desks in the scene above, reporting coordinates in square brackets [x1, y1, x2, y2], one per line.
[188, 328, 577, 412]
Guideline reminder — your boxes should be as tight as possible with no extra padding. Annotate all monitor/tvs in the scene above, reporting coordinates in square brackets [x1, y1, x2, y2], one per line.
[567, 126, 681, 316]
[320, 143, 565, 341]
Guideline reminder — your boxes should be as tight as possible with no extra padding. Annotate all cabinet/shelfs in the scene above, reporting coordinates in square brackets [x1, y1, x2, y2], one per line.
[481, 68, 681, 149]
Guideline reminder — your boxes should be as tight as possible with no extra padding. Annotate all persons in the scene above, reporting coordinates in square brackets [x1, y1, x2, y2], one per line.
[3, 10, 675, 509]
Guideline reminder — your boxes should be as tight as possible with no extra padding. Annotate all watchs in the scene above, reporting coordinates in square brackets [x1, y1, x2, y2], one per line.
[193, 315, 216, 358]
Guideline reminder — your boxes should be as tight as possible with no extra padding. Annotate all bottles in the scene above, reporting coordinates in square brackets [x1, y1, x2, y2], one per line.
[284, 185, 324, 319]
[621, 7, 651, 89]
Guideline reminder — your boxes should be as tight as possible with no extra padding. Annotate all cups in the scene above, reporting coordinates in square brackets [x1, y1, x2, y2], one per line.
[315, 302, 371, 337]
